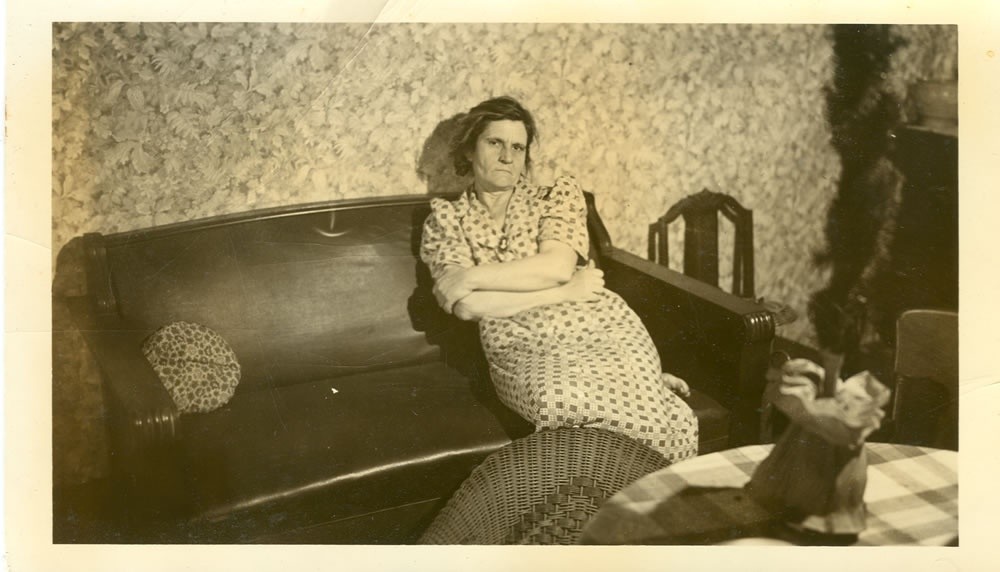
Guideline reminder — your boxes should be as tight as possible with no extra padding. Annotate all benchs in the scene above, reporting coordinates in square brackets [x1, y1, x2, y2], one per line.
[66, 189, 775, 545]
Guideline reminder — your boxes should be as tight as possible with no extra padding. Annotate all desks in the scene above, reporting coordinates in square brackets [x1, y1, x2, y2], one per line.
[577, 442, 959, 546]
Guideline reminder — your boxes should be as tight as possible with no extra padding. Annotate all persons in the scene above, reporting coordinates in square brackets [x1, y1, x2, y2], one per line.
[419, 96, 698, 463]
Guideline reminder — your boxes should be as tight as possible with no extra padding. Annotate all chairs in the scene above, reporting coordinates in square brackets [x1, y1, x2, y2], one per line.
[648, 188, 820, 365]
[890, 310, 958, 451]
[417, 430, 672, 545]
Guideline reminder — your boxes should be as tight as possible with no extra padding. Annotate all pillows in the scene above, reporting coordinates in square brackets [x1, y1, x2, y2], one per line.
[141, 322, 242, 415]
